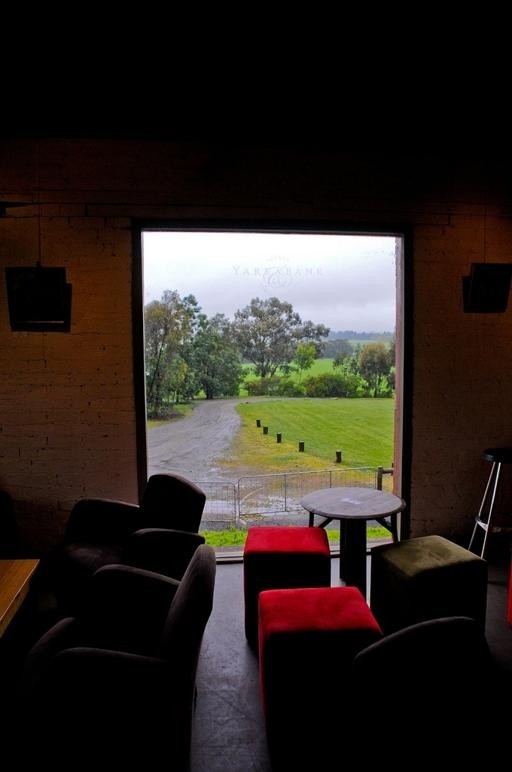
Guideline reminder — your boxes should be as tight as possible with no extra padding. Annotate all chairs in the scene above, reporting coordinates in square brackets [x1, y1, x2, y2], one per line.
[334, 616, 512, 772]
[21, 544, 215, 772]
[41, 473, 206, 608]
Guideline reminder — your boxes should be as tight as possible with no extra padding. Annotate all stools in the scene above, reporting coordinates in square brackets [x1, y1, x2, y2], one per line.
[371, 534, 487, 636]
[469, 444, 512, 573]
[245, 525, 330, 646]
[258, 587, 381, 766]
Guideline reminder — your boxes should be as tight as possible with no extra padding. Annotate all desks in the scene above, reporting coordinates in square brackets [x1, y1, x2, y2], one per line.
[302, 485, 406, 602]
[0, 558, 38, 636]
